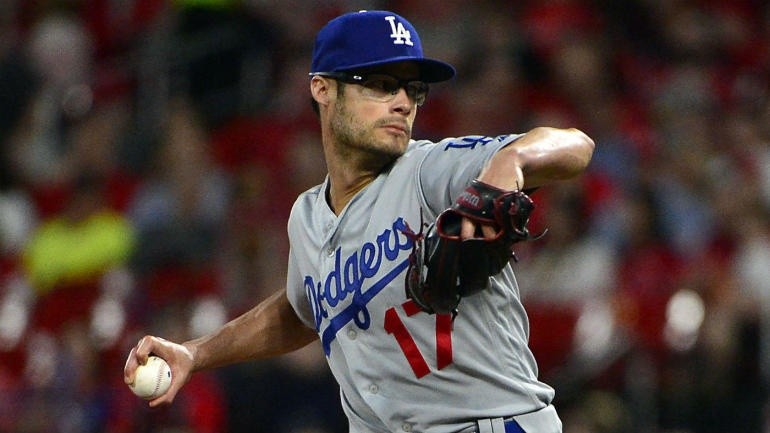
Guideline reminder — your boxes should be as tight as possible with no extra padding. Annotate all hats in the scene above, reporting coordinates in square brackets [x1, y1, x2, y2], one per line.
[310, 10, 456, 84]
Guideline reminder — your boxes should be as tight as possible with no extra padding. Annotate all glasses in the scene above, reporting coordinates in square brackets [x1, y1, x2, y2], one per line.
[308, 70, 431, 109]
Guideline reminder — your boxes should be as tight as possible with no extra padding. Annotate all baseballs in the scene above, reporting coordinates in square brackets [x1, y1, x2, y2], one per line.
[127, 355, 174, 402]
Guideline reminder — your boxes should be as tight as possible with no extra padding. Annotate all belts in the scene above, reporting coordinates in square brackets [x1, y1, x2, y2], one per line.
[504, 421, 525, 433]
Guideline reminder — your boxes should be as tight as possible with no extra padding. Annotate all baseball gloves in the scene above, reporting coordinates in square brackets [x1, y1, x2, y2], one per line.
[405, 178, 534, 317]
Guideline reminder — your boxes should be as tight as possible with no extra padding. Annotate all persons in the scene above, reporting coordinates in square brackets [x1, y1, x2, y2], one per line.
[0, 1, 350, 433]
[124, 8, 595, 433]
[352, 0, 770, 433]
